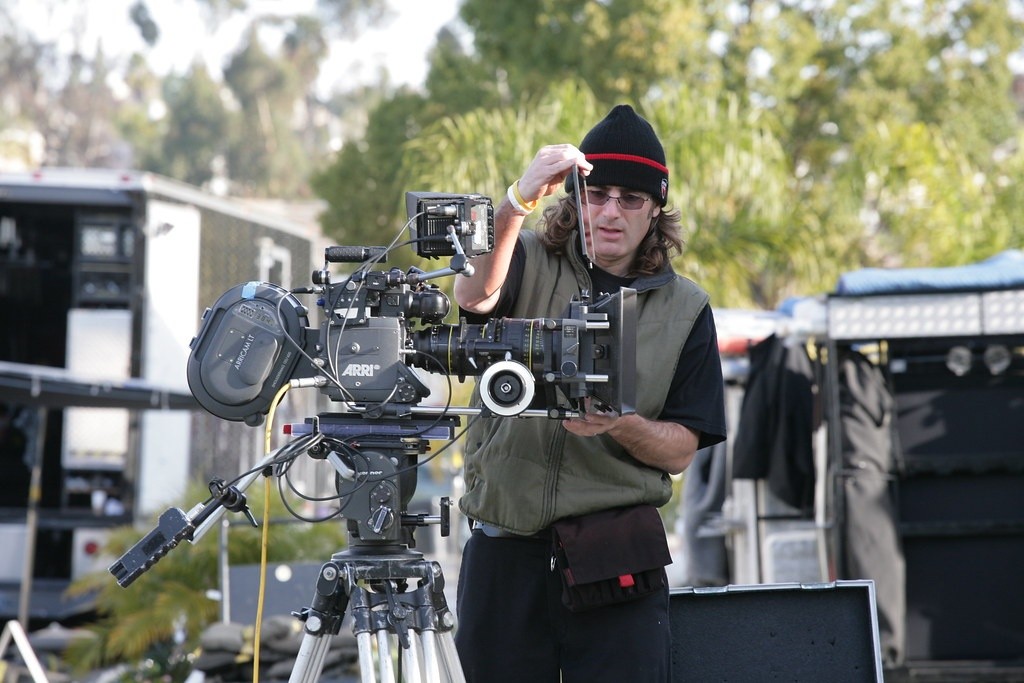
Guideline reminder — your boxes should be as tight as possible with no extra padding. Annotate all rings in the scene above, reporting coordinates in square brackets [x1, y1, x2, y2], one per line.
[593, 433, 596, 436]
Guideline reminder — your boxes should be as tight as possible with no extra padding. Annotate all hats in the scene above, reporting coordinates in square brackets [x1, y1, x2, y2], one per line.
[563, 105, 668, 207]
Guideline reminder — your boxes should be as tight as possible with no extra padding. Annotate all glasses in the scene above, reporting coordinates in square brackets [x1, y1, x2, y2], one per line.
[579, 188, 652, 209]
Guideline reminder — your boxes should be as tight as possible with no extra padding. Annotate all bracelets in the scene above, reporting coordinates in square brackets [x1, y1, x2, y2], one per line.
[508, 180, 537, 215]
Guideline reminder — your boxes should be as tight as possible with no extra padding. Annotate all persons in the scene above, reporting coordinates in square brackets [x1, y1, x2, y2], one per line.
[451, 105, 727, 683]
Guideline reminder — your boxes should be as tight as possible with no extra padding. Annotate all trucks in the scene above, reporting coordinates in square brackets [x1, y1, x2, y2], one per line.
[0, 167, 327, 629]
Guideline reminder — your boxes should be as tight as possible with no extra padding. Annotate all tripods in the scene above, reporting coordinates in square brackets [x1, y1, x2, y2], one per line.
[107, 407, 469, 683]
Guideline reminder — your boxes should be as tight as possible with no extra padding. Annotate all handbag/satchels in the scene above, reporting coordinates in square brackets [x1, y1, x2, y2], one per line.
[550, 502, 673, 610]
